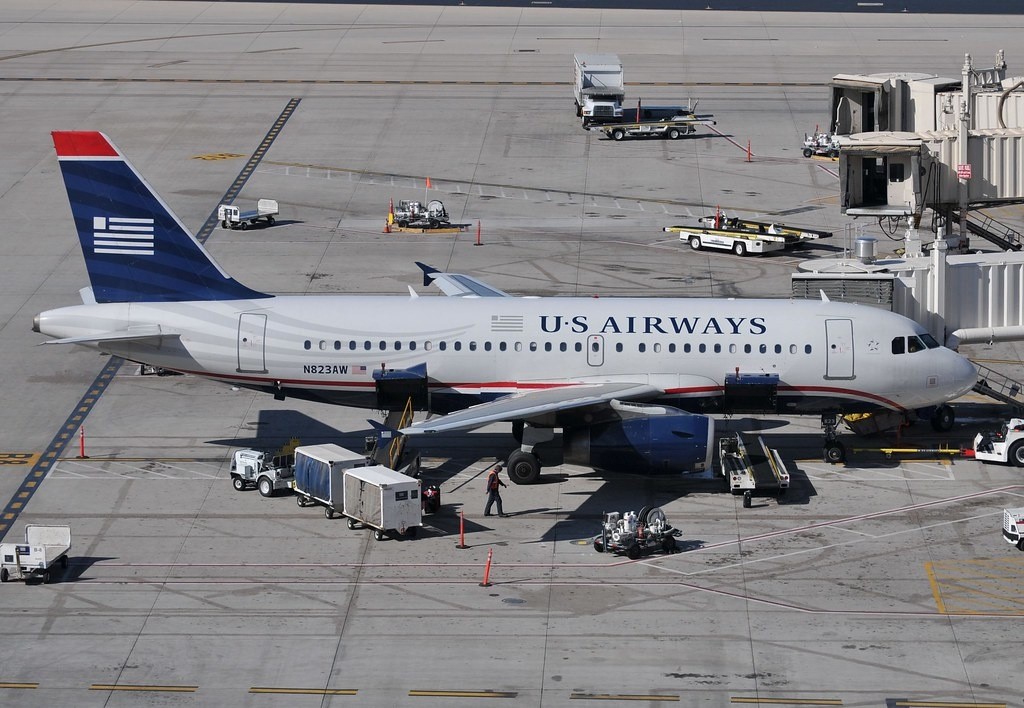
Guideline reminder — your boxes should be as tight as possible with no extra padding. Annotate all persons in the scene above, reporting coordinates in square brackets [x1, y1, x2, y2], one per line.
[484, 465, 509, 517]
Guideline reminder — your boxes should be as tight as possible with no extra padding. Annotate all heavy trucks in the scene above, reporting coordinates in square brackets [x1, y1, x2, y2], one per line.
[573, 52, 624, 127]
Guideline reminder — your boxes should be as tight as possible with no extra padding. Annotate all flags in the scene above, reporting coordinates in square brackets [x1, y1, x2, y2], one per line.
[426, 178, 432, 188]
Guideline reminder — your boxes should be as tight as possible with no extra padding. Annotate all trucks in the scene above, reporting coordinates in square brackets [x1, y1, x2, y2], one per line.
[228, 443, 424, 541]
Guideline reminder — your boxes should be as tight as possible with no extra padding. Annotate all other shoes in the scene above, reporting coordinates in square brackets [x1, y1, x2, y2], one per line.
[484, 514, 494, 516]
[499, 514, 508, 517]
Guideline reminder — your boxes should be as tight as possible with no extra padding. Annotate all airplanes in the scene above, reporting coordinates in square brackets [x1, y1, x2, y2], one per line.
[29, 128, 992, 465]
